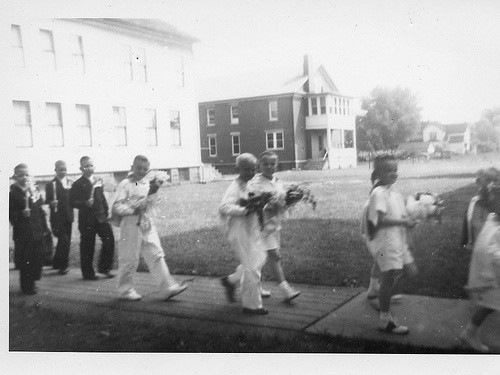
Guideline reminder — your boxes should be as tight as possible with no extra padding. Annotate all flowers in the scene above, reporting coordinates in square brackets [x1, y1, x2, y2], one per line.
[403, 191, 447, 228]
[137, 170, 171, 227]
[279, 183, 318, 212]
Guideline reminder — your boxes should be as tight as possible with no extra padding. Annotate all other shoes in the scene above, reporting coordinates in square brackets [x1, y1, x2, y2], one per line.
[82, 275, 97, 280]
[366, 294, 384, 311]
[244, 306, 269, 315]
[378, 318, 409, 334]
[160, 285, 189, 301]
[118, 289, 141, 300]
[459, 330, 488, 353]
[21, 285, 38, 294]
[98, 269, 115, 278]
[284, 290, 300, 301]
[59, 268, 69, 274]
[220, 278, 234, 303]
[258, 288, 272, 297]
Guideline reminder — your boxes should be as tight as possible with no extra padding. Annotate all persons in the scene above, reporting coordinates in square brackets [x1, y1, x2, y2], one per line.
[221, 152, 274, 316]
[252, 152, 300, 302]
[361, 154, 419, 335]
[461, 167, 500, 353]
[114, 155, 188, 300]
[46, 160, 74, 274]
[70, 157, 116, 280]
[9, 164, 54, 296]
